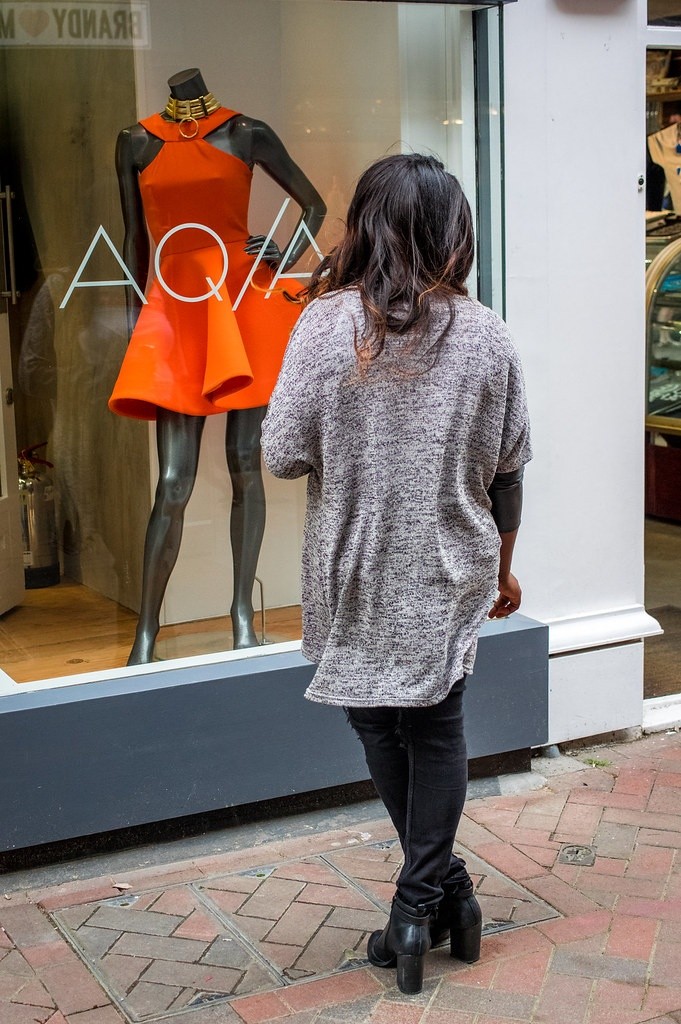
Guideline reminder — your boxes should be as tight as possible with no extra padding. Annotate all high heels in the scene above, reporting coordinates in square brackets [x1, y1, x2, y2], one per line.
[366, 892, 439, 994]
[429, 886, 483, 965]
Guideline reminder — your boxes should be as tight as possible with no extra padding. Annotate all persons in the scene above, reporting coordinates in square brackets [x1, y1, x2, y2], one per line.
[258, 149, 534, 996]
[113, 67, 330, 670]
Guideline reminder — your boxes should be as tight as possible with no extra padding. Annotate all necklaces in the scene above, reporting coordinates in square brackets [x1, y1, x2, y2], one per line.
[164, 91, 222, 139]
